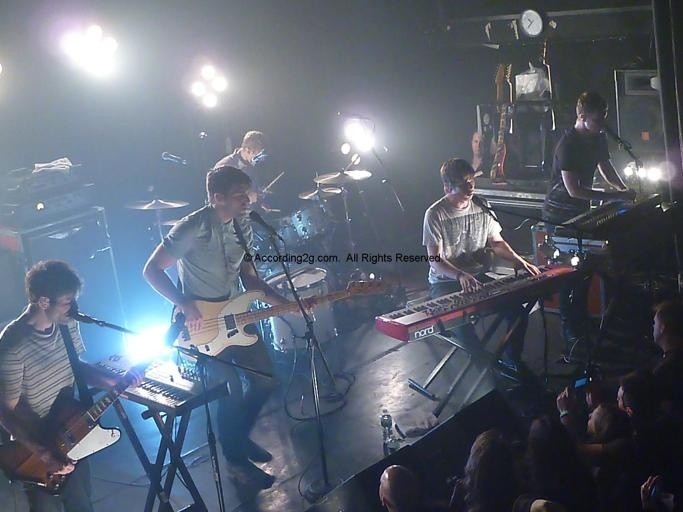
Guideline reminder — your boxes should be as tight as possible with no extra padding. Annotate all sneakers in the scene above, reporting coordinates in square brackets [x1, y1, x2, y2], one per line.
[243, 436, 272, 463]
[226, 460, 275, 490]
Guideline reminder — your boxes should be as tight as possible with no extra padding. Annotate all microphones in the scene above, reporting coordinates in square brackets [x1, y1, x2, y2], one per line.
[602, 124, 621, 142]
[249, 210, 277, 235]
[161, 151, 190, 165]
[197, 131, 207, 146]
[471, 196, 498, 221]
[70, 312, 95, 323]
[335, 111, 370, 122]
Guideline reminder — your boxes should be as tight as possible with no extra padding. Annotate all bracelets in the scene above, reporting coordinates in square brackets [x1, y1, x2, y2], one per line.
[560, 409, 570, 417]
[456, 270, 463, 280]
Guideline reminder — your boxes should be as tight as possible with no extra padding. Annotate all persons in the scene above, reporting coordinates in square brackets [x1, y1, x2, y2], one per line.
[144, 166, 318, 488]
[641, 474, 682, 512]
[423, 158, 540, 384]
[211, 130, 272, 216]
[0, 260, 145, 512]
[463, 427, 530, 512]
[587, 401, 629, 442]
[510, 495, 567, 512]
[379, 465, 421, 512]
[557, 370, 663, 466]
[528, 415, 577, 494]
[470, 132, 492, 176]
[651, 299, 683, 375]
[543, 92, 636, 359]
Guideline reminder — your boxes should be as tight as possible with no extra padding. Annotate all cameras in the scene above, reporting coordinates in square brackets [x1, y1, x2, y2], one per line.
[574, 377, 591, 388]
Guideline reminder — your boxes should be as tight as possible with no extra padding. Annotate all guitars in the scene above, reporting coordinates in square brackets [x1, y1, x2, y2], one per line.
[0, 362, 150, 496]
[171, 279, 377, 362]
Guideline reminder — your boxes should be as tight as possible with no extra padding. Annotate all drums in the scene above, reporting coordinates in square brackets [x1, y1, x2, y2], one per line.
[268, 218, 300, 253]
[263, 267, 335, 351]
[291, 203, 330, 243]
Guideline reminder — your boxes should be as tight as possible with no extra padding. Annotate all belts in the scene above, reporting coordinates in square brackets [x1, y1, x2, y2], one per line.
[543, 203, 569, 215]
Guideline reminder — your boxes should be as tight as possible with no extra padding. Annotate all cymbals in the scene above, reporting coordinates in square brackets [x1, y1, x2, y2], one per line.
[314, 171, 373, 185]
[125, 198, 189, 210]
[153, 221, 177, 226]
[298, 187, 342, 200]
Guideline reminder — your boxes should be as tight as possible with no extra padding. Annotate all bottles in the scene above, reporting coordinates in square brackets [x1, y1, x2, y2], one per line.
[380, 409, 393, 443]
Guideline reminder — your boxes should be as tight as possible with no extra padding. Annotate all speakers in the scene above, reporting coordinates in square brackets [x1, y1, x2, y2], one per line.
[1, 206, 134, 365]
[615, 68, 671, 195]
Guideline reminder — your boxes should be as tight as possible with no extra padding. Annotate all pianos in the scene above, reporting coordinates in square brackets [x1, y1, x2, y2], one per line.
[89, 354, 231, 418]
[375, 266, 580, 343]
[555, 192, 661, 241]
